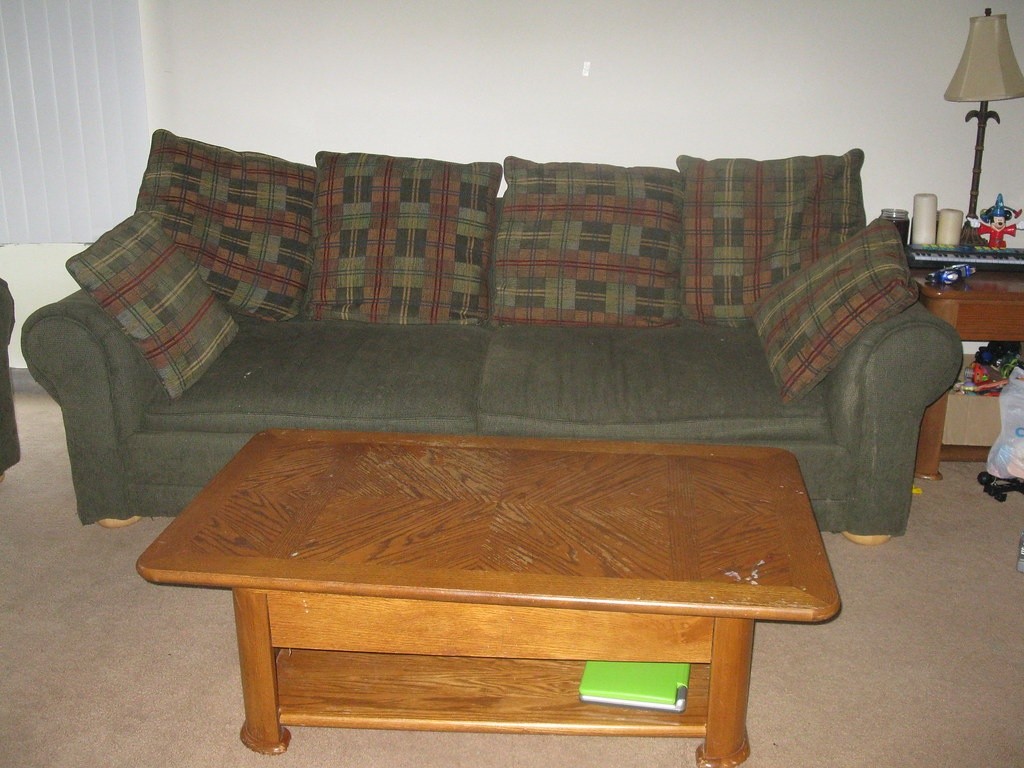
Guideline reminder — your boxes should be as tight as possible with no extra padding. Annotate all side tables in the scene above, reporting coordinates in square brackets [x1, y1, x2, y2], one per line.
[910, 267, 1024, 482]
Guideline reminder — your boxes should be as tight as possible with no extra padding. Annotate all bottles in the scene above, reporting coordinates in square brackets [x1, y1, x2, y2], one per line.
[879, 209, 909, 246]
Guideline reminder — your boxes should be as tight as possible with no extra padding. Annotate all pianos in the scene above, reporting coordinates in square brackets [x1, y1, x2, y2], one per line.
[905, 243, 1024, 274]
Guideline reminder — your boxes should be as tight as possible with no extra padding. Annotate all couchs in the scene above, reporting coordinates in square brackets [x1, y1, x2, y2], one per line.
[22, 285, 963, 545]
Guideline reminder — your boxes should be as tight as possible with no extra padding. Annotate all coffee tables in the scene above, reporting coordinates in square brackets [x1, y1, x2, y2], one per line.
[135, 428, 840, 768]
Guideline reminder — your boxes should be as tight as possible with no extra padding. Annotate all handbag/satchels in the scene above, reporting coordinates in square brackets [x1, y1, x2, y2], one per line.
[986, 367, 1024, 479]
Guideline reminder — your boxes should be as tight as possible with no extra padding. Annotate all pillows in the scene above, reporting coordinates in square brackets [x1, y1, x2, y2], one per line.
[134, 129, 317, 322]
[489, 156, 684, 326]
[65, 212, 240, 398]
[747, 219, 919, 403]
[304, 151, 503, 326]
[677, 148, 867, 329]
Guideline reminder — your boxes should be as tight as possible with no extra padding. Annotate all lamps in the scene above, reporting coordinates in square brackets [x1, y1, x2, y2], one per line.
[945, 9, 1024, 245]
